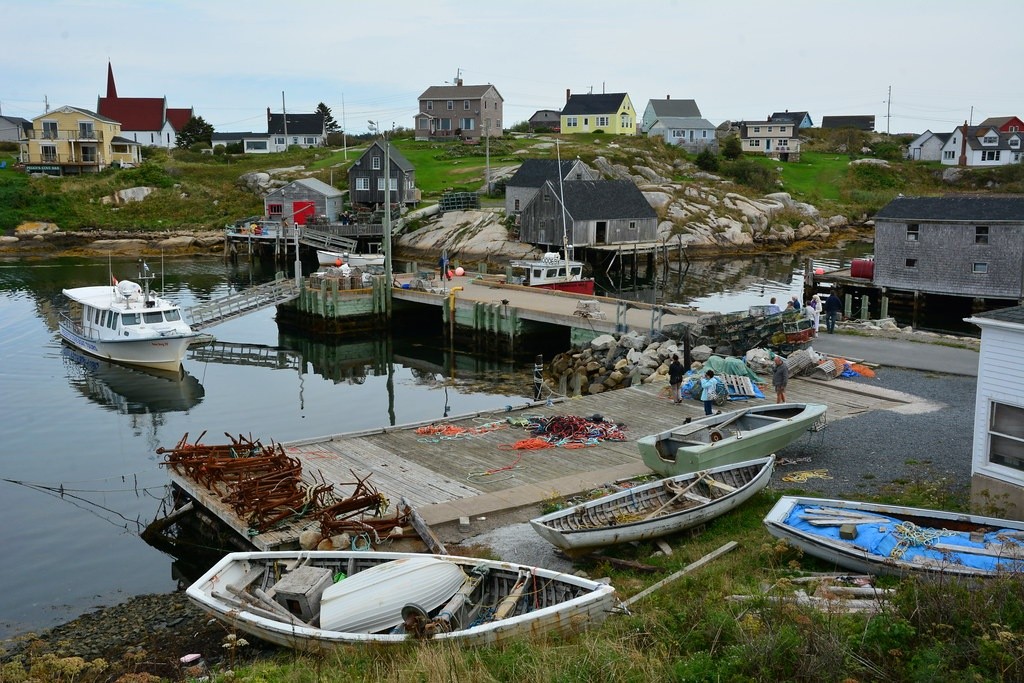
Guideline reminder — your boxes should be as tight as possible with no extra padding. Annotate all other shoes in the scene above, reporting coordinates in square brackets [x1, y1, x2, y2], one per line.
[828, 331, 830, 334]
[816, 333, 818, 338]
[831, 331, 834, 334]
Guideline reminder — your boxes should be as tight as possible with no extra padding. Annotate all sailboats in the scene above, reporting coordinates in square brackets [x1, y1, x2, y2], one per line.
[496, 138, 599, 299]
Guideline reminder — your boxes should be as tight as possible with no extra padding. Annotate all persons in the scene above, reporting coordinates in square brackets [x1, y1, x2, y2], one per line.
[771, 357, 788, 403]
[700, 371, 716, 415]
[770, 292, 840, 338]
[341, 211, 354, 226]
[669, 356, 684, 404]
[439, 255, 452, 281]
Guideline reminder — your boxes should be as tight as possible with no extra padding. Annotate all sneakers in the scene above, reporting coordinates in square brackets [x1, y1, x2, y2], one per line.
[679, 399, 683, 403]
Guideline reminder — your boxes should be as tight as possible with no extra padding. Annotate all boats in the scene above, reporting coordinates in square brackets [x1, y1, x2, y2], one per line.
[58, 339, 206, 440]
[529, 453, 776, 550]
[316, 249, 386, 269]
[185, 552, 616, 658]
[760, 493, 1023, 587]
[636, 401, 829, 480]
[56, 247, 203, 374]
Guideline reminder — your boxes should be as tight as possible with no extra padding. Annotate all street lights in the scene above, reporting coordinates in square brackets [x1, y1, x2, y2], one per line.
[479, 125, 492, 194]
[367, 124, 391, 319]
